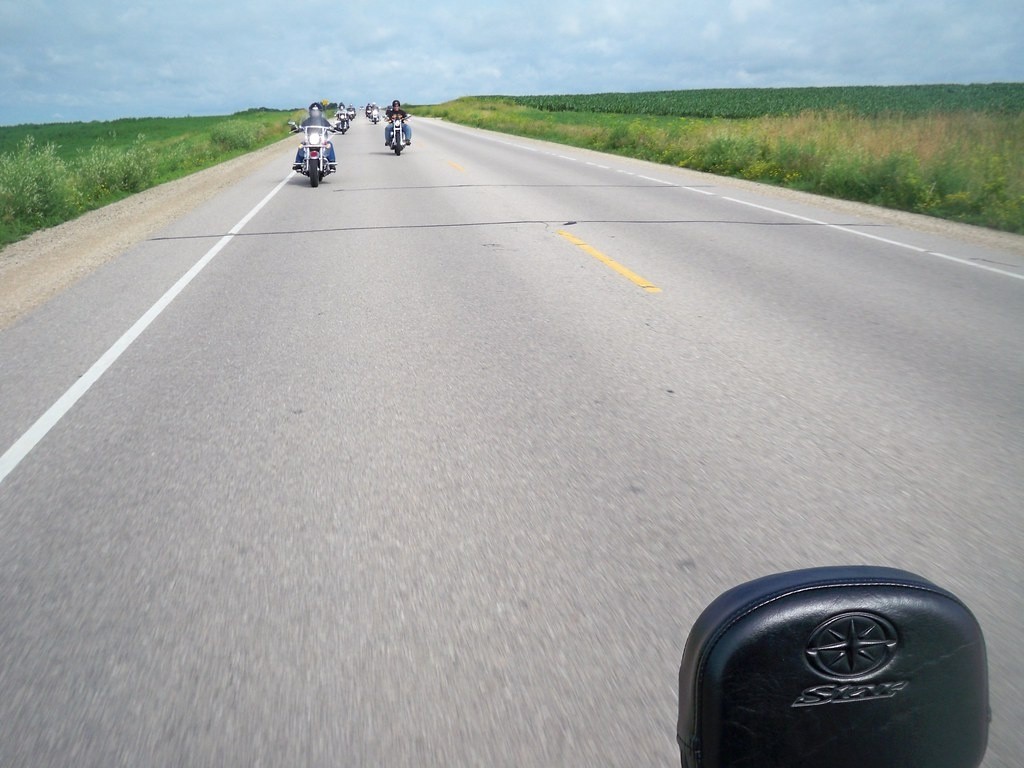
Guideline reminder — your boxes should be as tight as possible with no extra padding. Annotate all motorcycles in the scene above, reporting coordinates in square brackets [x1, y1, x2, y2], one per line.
[287, 110, 346, 188]
[347, 110, 356, 122]
[382, 114, 412, 156]
[333, 111, 352, 135]
[366, 109, 372, 119]
[369, 108, 380, 125]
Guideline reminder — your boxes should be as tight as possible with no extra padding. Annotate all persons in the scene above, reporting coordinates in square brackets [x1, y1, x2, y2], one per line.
[292, 103, 336, 171]
[385, 100, 411, 146]
[335, 103, 356, 129]
[359, 101, 381, 120]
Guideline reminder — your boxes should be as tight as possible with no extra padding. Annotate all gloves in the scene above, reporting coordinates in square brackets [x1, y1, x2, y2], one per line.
[331, 128, 340, 133]
[291, 125, 299, 134]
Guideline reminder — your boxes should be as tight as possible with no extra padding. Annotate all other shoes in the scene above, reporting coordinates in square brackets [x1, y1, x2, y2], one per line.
[406, 140, 411, 145]
[330, 165, 335, 169]
[293, 165, 302, 170]
[385, 142, 391, 146]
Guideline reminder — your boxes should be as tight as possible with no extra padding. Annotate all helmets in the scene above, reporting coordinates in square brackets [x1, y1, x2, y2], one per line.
[350, 104, 352, 105]
[309, 103, 322, 117]
[368, 104, 370, 105]
[339, 104, 345, 109]
[372, 102, 376, 105]
[393, 100, 400, 107]
